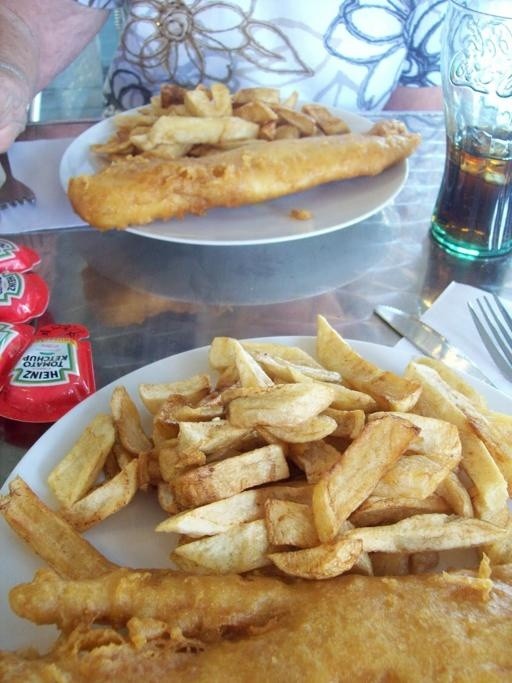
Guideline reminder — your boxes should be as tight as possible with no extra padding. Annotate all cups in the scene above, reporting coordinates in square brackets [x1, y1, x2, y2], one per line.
[430, 0, 512, 260]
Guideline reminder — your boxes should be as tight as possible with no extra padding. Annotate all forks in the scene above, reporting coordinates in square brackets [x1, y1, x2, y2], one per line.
[0, 151, 36, 210]
[466, 291, 512, 383]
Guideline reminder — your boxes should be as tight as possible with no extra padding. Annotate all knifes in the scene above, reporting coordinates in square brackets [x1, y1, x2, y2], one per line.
[373, 304, 496, 389]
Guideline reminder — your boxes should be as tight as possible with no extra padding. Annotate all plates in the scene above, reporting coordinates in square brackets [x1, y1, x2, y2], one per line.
[59, 98, 410, 247]
[0, 335, 512, 656]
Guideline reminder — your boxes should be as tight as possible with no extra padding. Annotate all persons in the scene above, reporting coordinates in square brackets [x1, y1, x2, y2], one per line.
[0, 1, 475, 155]
